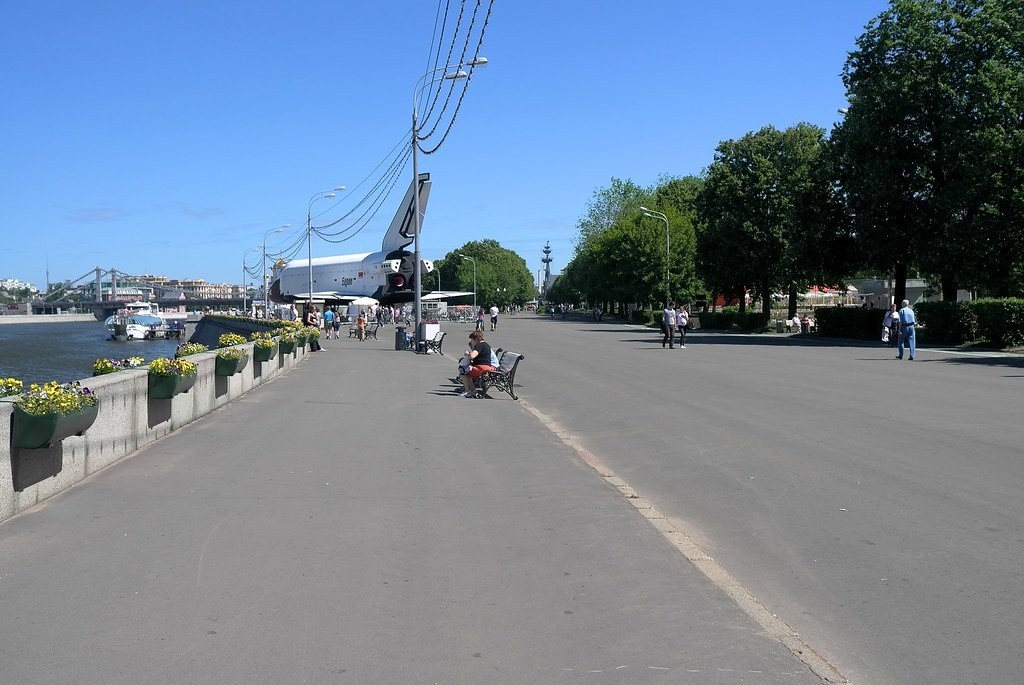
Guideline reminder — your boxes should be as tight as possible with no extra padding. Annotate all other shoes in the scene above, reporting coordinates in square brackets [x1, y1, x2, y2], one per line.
[335, 334, 338, 339]
[318, 347, 328, 352]
[470, 389, 477, 397]
[328, 336, 332, 340]
[680, 345, 686, 348]
[662, 341, 665, 347]
[359, 338, 361, 341]
[896, 355, 902, 359]
[894, 345, 899, 348]
[457, 390, 472, 397]
[908, 357, 913, 360]
[670, 346, 675, 349]
[891, 345, 893, 348]
[326, 336, 329, 339]
[337, 335, 340, 339]
[456, 377, 464, 384]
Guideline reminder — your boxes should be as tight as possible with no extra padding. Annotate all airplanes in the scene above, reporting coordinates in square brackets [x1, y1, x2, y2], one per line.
[267, 172, 476, 305]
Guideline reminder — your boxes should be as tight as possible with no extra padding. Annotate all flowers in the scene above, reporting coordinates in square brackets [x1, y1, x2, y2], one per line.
[218, 347, 248, 359]
[93, 356, 144, 373]
[0, 376, 23, 398]
[176, 341, 209, 356]
[149, 358, 198, 377]
[218, 332, 247, 346]
[16, 380, 96, 415]
[249, 319, 320, 348]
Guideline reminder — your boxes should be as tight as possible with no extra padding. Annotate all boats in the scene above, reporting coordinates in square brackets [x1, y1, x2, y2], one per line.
[104, 309, 170, 340]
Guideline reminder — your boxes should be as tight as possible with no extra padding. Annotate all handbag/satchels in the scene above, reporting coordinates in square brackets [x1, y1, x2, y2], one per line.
[882, 326, 890, 342]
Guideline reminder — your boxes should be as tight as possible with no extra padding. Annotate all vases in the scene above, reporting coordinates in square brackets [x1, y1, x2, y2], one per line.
[149, 370, 196, 398]
[254, 345, 278, 361]
[12, 400, 98, 448]
[311, 334, 320, 343]
[298, 337, 309, 347]
[280, 341, 298, 354]
[216, 356, 248, 376]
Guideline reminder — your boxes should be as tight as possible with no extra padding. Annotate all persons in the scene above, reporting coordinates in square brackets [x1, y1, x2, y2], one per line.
[304, 302, 328, 352]
[884, 304, 901, 348]
[455, 329, 500, 399]
[675, 306, 689, 349]
[896, 300, 916, 360]
[324, 306, 341, 339]
[393, 304, 417, 327]
[792, 313, 811, 333]
[476, 308, 486, 331]
[661, 302, 677, 349]
[489, 303, 499, 332]
[503, 303, 522, 315]
[257, 307, 263, 318]
[592, 306, 603, 322]
[290, 304, 298, 322]
[356, 303, 394, 342]
[550, 303, 574, 320]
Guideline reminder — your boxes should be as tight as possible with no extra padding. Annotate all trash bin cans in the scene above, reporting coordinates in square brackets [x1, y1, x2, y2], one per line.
[395, 326, 406, 350]
[776, 319, 783, 333]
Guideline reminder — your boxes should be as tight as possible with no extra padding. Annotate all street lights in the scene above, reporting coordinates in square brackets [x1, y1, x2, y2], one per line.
[264, 224, 292, 318]
[409, 56, 491, 358]
[308, 186, 347, 303]
[242, 244, 264, 312]
[458, 253, 477, 309]
[639, 206, 671, 308]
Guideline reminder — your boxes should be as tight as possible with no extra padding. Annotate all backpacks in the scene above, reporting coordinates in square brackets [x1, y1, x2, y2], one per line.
[458, 352, 473, 375]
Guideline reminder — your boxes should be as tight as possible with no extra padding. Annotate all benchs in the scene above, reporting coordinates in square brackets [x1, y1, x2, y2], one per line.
[349, 322, 379, 341]
[421, 332, 446, 356]
[459, 347, 524, 400]
[365, 323, 379, 340]
